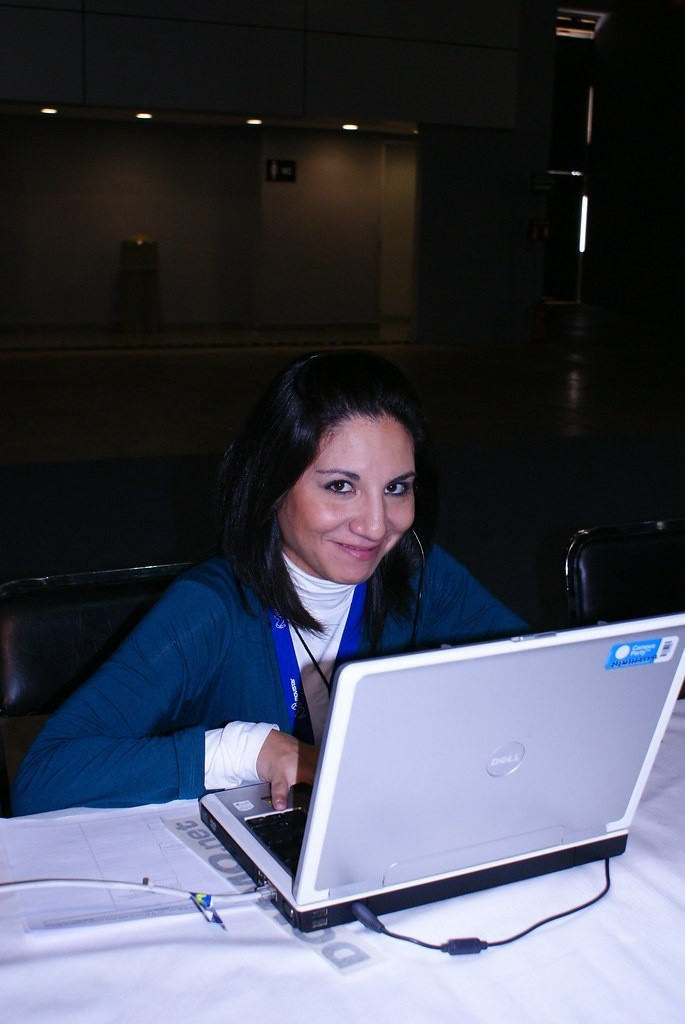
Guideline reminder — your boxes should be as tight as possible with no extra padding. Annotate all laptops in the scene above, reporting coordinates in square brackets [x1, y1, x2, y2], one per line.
[198, 608, 685, 930]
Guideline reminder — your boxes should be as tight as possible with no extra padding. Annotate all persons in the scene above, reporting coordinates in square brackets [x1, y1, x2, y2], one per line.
[8, 349, 536, 817]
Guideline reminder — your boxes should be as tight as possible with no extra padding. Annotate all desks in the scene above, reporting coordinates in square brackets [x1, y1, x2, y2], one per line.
[0, 697, 685, 1024]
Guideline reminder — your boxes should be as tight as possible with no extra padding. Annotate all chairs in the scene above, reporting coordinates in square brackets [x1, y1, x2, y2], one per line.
[0, 560, 194, 820]
[561, 519, 685, 623]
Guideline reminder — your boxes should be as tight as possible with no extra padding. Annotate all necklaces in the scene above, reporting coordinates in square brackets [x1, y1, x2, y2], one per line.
[291, 625, 330, 690]
